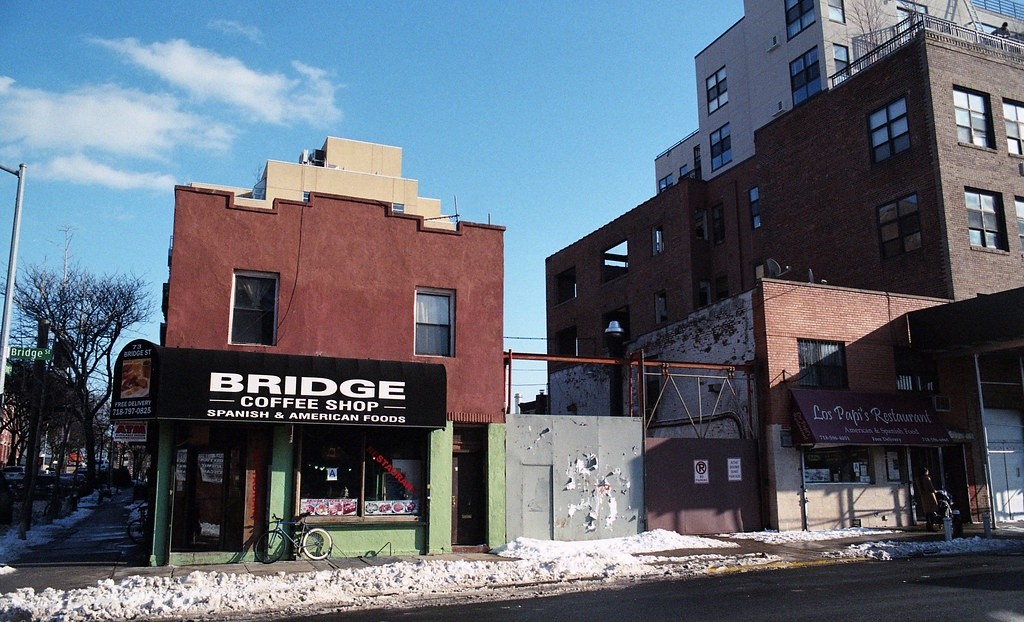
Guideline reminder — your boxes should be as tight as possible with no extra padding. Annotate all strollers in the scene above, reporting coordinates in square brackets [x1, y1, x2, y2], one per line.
[933, 488, 953, 529]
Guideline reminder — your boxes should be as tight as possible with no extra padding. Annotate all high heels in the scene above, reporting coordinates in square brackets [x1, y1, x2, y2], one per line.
[926, 523, 938, 533]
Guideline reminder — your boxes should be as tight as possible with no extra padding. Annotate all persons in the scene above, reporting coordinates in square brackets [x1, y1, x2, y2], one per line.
[991, 22, 1010, 50]
[916, 467, 940, 532]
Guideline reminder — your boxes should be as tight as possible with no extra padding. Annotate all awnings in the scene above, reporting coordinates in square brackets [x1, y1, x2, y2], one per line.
[789, 389, 954, 449]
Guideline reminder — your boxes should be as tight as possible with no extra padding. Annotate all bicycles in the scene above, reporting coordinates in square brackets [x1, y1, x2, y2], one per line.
[129, 500, 148, 539]
[255, 512, 333, 564]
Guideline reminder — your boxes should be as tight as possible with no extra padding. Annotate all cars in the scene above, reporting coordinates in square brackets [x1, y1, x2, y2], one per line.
[3, 458, 109, 491]
